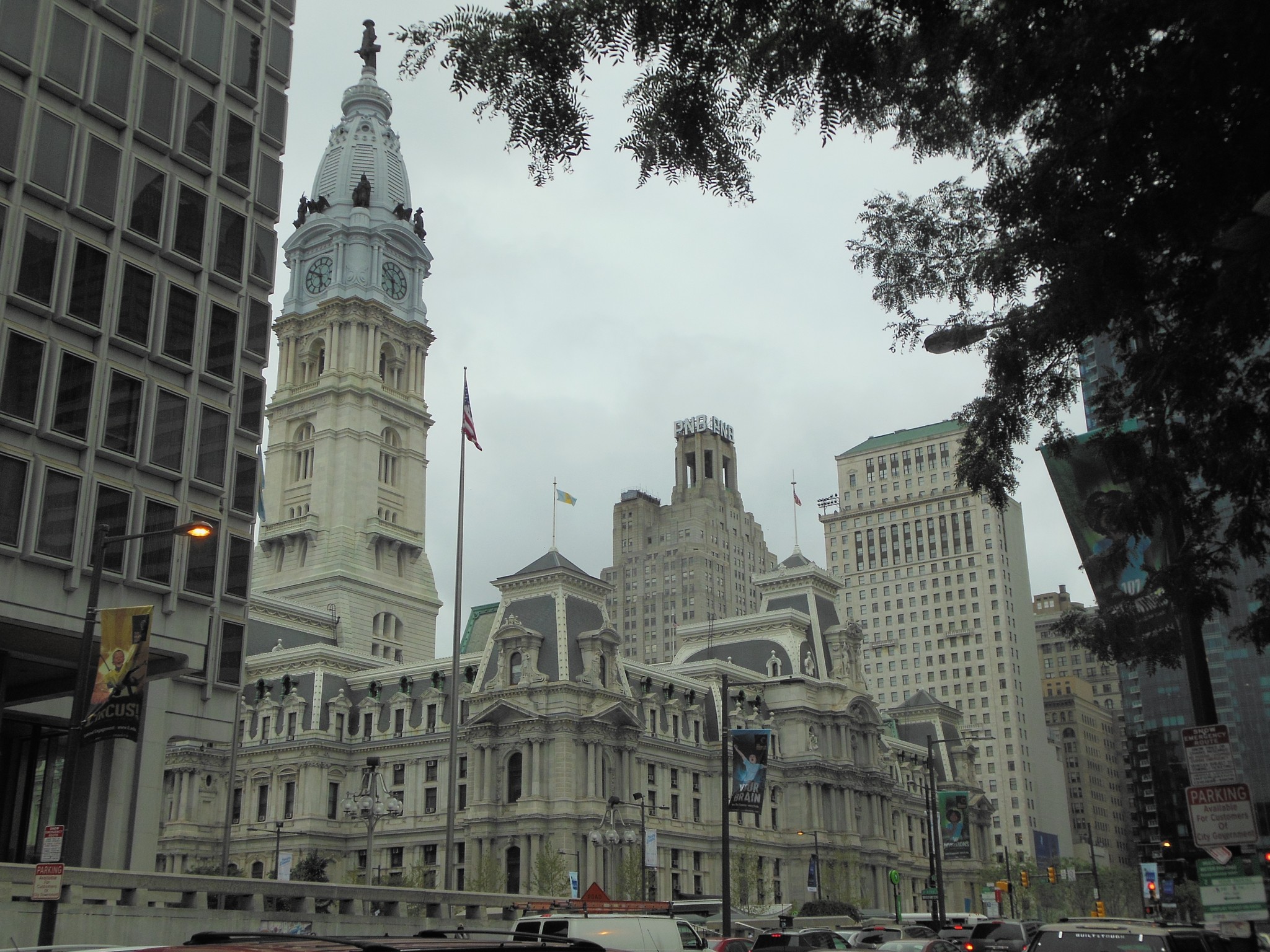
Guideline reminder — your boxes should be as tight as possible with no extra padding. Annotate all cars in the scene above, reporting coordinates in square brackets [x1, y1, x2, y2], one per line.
[91, 908, 1270, 952]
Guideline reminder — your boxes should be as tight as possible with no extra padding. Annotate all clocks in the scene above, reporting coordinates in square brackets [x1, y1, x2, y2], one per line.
[382, 259, 410, 303]
[302, 254, 333, 297]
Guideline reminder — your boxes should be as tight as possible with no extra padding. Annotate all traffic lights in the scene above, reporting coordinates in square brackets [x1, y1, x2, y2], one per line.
[1095, 901, 1106, 918]
[1047, 866, 1057, 884]
[1148, 882, 1157, 905]
[1019, 870, 1030, 888]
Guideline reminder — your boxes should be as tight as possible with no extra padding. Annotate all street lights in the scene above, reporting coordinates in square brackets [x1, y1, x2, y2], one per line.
[246, 822, 308, 910]
[796, 830, 822, 902]
[588, 803, 637, 902]
[339, 763, 405, 916]
[50, 520, 216, 872]
[607, 792, 670, 902]
[926, 733, 996, 928]
[719, 674, 806, 942]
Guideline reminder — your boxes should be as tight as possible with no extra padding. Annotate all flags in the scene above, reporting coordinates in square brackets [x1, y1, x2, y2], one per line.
[556, 489, 577, 507]
[793, 488, 802, 507]
[462, 375, 482, 452]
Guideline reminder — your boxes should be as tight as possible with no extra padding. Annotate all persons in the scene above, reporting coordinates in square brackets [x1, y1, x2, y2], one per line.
[292, 171, 426, 243]
[354, 20, 376, 69]
